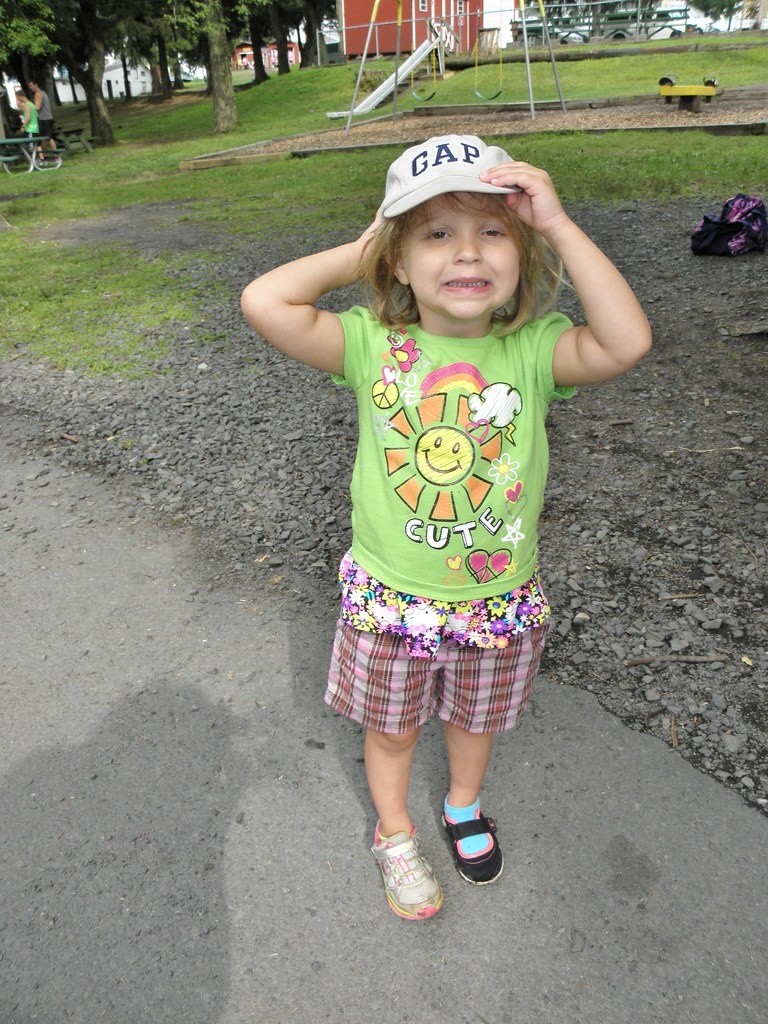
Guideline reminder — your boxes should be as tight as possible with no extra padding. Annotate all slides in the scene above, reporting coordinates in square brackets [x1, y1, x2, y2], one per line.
[323, 33, 440, 123]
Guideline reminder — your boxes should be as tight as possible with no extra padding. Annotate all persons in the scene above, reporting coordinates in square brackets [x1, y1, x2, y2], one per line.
[239, 135, 653, 922]
[14, 79, 63, 164]
[237, 52, 294, 71]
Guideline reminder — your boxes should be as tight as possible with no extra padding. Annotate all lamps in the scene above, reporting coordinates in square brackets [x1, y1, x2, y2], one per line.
[659, 73, 678, 86]
[703, 76, 720, 88]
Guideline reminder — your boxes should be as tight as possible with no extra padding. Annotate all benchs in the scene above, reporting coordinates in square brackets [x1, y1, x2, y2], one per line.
[0, 135, 98, 175]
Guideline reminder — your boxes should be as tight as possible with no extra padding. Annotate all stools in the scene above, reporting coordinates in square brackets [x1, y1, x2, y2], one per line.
[660, 85, 716, 111]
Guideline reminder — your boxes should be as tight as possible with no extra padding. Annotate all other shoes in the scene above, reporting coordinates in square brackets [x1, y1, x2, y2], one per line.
[51, 157, 60, 162]
[36, 159, 47, 164]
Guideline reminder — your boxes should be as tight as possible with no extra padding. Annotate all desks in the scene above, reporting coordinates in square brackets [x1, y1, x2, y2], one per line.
[7, 128, 91, 161]
[0, 137, 49, 171]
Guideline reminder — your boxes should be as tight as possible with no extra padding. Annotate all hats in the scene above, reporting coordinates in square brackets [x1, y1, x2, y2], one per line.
[378, 134, 524, 219]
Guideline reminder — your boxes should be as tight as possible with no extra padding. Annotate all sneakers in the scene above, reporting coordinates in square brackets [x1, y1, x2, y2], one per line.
[440, 811, 504, 885]
[371, 818, 445, 921]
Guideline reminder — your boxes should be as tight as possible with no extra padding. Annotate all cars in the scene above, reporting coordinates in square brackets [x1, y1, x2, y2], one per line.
[515, 24, 583, 45]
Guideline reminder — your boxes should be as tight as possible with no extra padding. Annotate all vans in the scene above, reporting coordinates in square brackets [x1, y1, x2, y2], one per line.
[604, 7, 701, 43]
